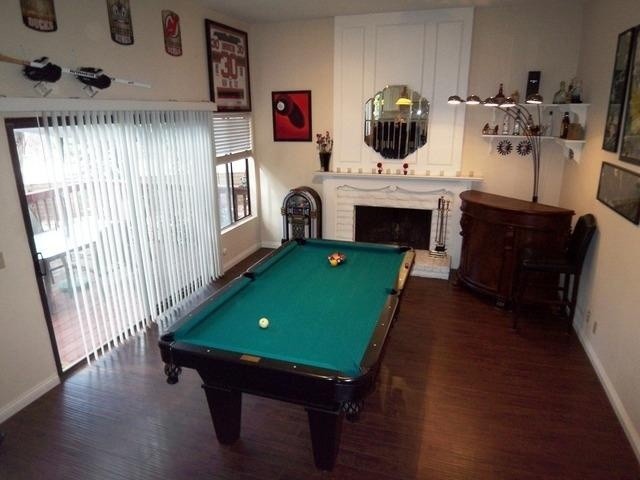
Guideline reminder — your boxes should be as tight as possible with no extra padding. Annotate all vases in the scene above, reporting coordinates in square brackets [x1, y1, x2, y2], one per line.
[318, 153, 331, 171]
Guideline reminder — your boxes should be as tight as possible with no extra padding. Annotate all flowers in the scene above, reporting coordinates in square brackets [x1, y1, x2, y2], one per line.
[317, 131, 332, 153]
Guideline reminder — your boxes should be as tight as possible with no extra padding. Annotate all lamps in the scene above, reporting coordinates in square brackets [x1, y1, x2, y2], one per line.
[448, 94, 542, 203]
[22, 56, 151, 99]
[396, 87, 411, 104]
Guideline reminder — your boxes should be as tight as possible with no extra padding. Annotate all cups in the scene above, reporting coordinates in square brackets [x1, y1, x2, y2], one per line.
[319, 165, 475, 178]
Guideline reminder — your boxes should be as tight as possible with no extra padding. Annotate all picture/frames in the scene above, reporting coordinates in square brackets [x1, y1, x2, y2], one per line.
[205, 18, 252, 112]
[602, 25, 640, 166]
[596, 160, 640, 225]
[271, 90, 311, 142]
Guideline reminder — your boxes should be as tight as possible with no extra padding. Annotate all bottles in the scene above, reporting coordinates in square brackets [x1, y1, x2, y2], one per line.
[496, 82, 507, 103]
[552, 74, 583, 105]
[503, 106, 570, 139]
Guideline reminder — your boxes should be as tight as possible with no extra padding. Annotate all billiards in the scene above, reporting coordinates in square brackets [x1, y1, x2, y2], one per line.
[328, 252, 346, 266]
[258, 317, 269, 328]
[343, 402, 358, 416]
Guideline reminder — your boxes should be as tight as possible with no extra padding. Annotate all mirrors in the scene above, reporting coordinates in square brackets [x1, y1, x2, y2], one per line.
[364, 85, 429, 159]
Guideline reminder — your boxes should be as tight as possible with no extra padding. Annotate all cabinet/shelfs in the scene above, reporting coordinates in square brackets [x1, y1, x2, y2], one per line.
[481, 103, 589, 144]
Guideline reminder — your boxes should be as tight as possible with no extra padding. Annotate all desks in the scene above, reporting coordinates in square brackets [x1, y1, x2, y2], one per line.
[453, 190, 576, 312]
[33, 217, 96, 290]
[157, 238, 416, 471]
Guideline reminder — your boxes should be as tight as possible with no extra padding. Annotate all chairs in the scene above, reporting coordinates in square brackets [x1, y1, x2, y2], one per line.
[512, 213, 595, 329]
[37, 252, 56, 323]
[28, 208, 43, 235]
